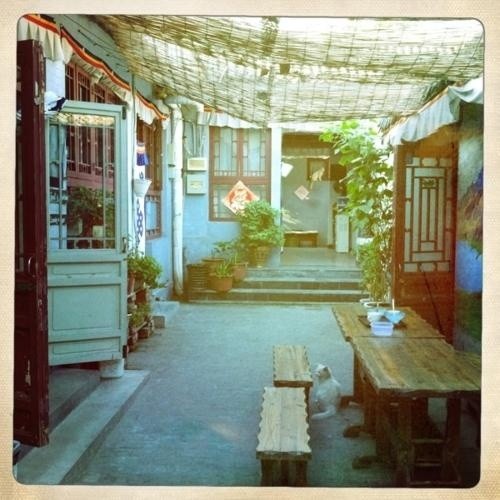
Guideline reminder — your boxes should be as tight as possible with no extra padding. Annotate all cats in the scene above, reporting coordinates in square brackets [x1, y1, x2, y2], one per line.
[310, 363, 343, 420]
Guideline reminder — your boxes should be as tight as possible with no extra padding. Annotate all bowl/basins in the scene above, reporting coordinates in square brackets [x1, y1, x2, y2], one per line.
[367, 307, 406, 324]
[369, 321, 394, 337]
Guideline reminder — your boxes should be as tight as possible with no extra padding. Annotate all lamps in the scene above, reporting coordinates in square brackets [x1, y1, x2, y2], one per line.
[133, 172, 153, 197]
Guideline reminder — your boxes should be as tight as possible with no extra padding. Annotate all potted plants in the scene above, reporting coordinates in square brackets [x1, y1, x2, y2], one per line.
[67, 187, 114, 238]
[126, 255, 173, 353]
[185, 198, 289, 293]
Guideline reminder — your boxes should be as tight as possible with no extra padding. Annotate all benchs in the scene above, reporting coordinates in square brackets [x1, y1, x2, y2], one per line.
[255, 343, 315, 488]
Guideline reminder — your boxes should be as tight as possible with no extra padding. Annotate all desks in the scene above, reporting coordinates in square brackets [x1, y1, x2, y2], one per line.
[330, 305, 480, 488]
